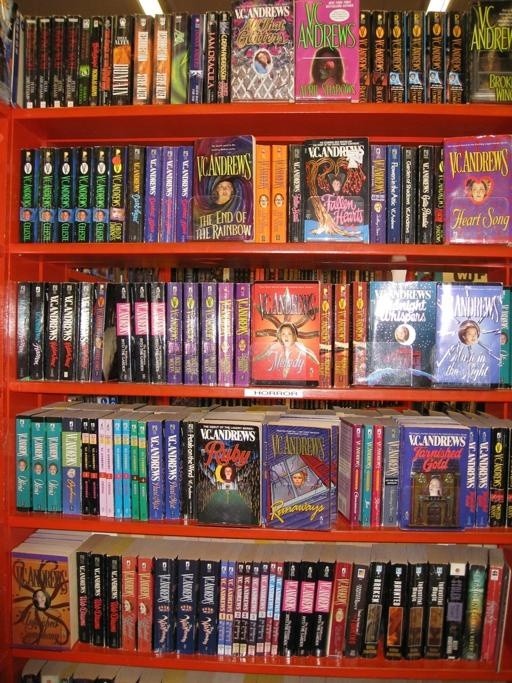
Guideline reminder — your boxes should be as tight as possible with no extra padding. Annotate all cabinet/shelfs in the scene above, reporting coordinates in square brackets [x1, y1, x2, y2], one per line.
[0, 102, 512, 682]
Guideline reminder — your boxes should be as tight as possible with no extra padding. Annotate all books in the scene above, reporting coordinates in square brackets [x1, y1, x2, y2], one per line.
[20, 134, 512, 245]
[10, 527, 512, 683]
[20, 265, 512, 389]
[1, 1, 512, 108]
[16, 394, 512, 531]
